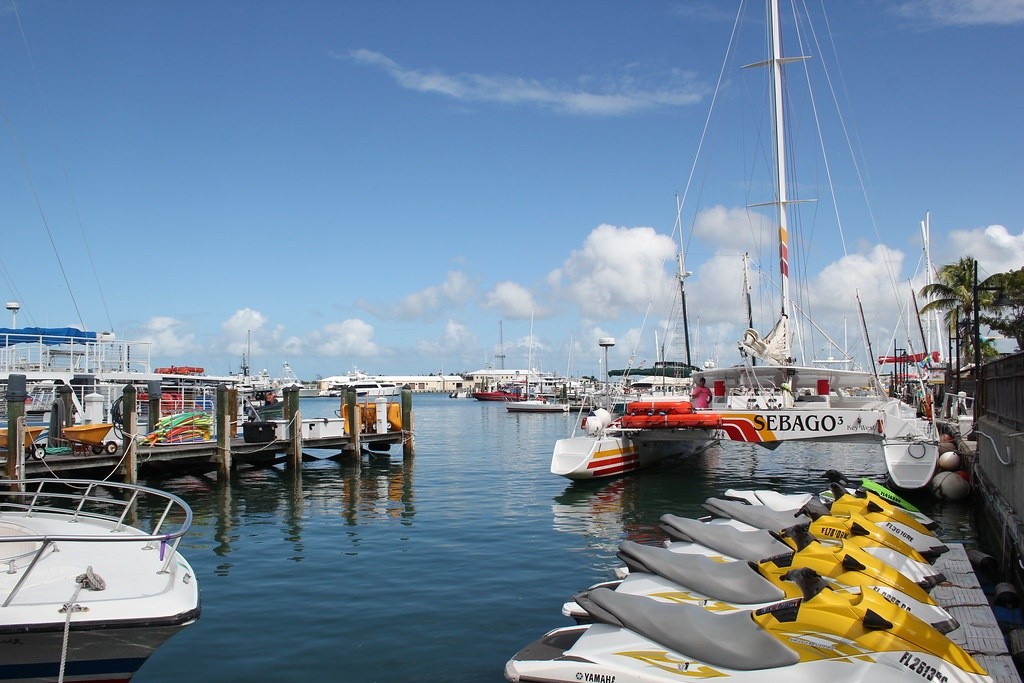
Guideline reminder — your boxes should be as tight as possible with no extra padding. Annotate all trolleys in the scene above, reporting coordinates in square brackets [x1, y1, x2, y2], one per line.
[340, 389, 403, 435]
[44, 423, 117, 457]
[0, 426, 46, 460]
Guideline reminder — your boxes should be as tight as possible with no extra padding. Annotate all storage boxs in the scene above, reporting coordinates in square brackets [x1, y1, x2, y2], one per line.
[242, 422, 278, 443]
[267, 418, 346, 441]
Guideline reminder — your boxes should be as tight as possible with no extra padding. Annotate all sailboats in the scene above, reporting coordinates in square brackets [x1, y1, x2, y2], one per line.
[239, 330, 276, 408]
[608, 190, 758, 414]
[547, 0, 943, 489]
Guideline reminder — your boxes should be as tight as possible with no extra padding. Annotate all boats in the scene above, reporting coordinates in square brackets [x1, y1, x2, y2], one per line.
[448, 391, 458, 398]
[472, 384, 546, 401]
[317, 366, 402, 396]
[506, 384, 606, 411]
[501, 469, 999, 683]
[0, 477, 201, 666]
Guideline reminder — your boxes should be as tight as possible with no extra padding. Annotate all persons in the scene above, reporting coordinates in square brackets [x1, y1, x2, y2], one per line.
[692, 378, 712, 408]
[958, 389, 967, 410]
[903, 384, 923, 400]
[265, 392, 278, 405]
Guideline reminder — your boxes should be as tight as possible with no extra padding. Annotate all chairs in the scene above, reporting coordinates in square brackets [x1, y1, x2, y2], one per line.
[766, 399, 782, 409]
[746, 399, 759, 409]
